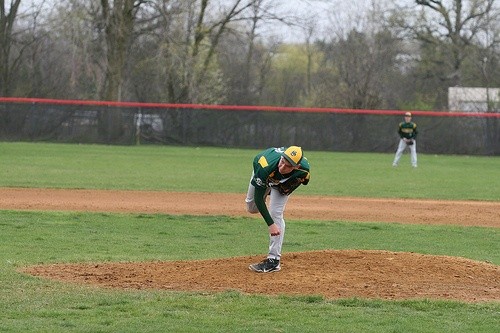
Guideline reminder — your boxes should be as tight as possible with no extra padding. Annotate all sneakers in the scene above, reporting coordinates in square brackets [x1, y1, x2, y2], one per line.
[248, 258, 281, 273]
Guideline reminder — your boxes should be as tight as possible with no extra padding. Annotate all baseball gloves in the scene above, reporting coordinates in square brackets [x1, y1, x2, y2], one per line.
[405, 140, 414, 145]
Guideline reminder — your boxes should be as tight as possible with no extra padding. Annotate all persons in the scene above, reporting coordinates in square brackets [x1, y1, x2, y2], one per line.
[245, 146, 311, 273]
[393, 112, 418, 167]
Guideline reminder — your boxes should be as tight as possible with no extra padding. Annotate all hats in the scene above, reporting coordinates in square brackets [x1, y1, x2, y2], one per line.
[282, 145, 303, 166]
[404, 112, 412, 116]
